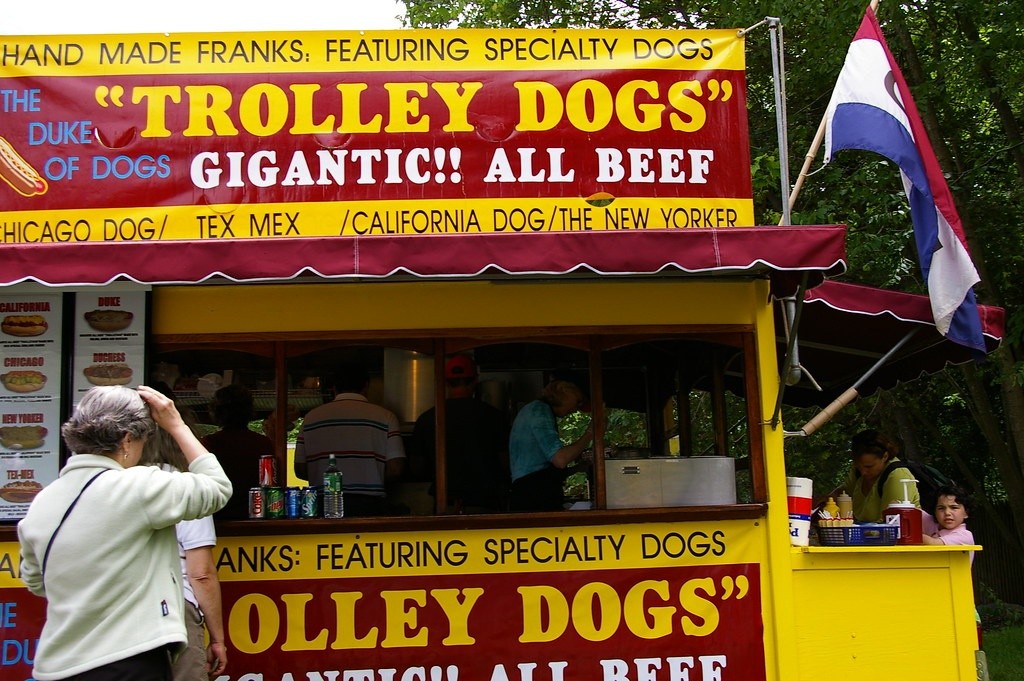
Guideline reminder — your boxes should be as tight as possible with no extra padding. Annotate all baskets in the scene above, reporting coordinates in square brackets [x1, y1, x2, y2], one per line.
[816, 524, 899, 547]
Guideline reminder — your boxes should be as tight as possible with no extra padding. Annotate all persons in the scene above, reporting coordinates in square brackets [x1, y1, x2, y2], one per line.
[136, 410, 227, 681]
[17, 384, 234, 681]
[197, 386, 278, 520]
[407, 356, 512, 515]
[508, 380, 609, 512]
[920, 485, 989, 681]
[294, 362, 407, 517]
[811, 430, 922, 528]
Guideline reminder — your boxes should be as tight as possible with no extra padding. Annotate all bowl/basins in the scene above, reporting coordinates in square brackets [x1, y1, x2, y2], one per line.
[0, 311, 134, 503]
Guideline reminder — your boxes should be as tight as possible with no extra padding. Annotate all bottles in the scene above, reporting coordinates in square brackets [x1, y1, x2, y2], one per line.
[837, 490, 853, 518]
[883, 504, 923, 544]
[824, 496, 840, 519]
[323, 459, 344, 519]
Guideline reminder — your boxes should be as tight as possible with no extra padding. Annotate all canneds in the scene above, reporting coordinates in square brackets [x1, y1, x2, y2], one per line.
[249, 486, 318, 520]
[258, 455, 278, 486]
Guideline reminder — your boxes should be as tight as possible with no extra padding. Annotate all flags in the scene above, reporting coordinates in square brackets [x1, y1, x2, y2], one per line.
[824, 6, 986, 353]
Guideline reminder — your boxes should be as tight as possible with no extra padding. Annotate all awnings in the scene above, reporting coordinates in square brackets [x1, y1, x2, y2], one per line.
[782, 280, 1004, 408]
[0, 222, 848, 286]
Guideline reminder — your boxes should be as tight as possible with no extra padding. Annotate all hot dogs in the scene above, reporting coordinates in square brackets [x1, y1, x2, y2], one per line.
[84, 309, 133, 331]
[0, 371, 47, 392]
[0, 481, 44, 503]
[0, 426, 48, 449]
[83, 365, 133, 386]
[1, 315, 48, 336]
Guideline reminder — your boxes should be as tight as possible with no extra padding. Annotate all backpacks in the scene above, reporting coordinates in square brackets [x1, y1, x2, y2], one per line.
[877, 460, 958, 515]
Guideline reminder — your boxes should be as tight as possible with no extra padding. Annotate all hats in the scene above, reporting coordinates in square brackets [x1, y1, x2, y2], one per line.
[445, 354, 476, 380]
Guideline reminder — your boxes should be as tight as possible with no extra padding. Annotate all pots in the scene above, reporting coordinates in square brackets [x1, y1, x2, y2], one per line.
[613, 447, 652, 458]
